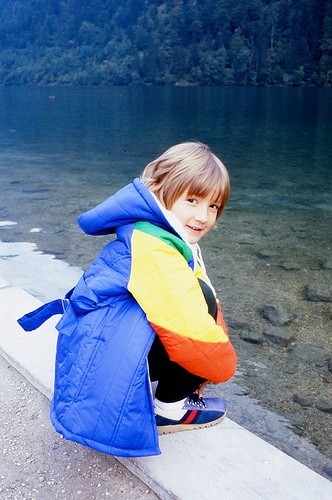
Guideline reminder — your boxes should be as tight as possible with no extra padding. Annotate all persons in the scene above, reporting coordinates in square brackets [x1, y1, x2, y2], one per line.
[19, 137, 238, 433]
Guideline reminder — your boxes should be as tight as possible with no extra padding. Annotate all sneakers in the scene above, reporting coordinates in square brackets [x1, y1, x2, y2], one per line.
[152, 394, 227, 435]
